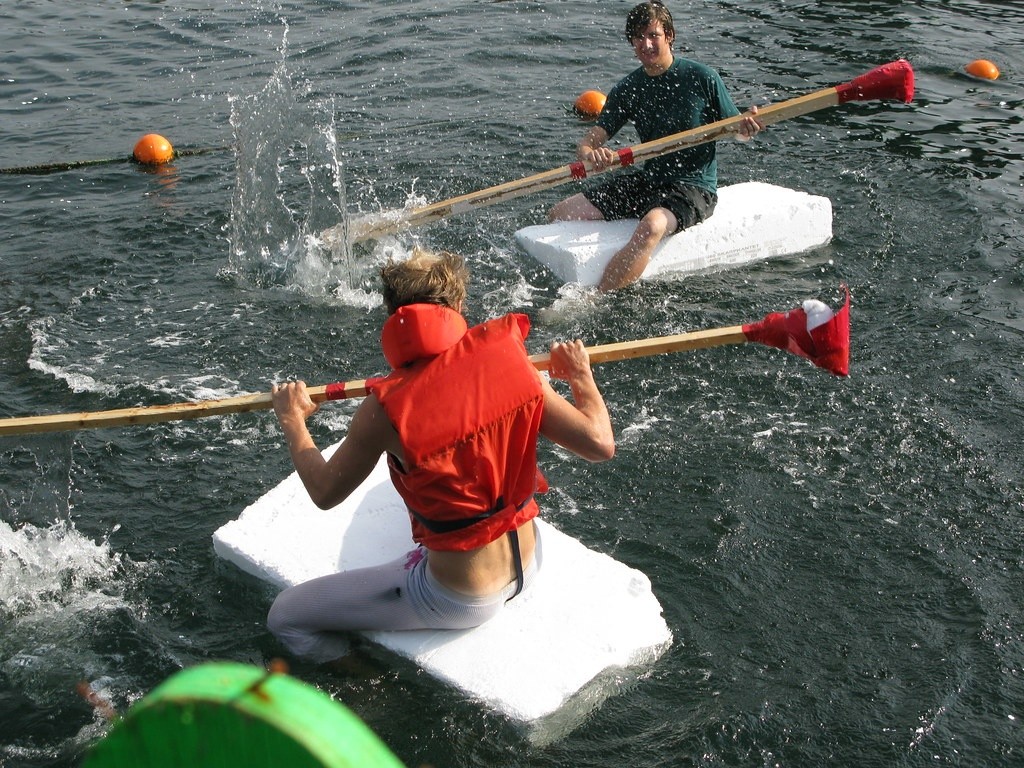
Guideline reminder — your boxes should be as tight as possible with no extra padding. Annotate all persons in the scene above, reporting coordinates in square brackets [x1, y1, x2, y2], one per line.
[547, 0, 766, 290]
[268, 248, 615, 666]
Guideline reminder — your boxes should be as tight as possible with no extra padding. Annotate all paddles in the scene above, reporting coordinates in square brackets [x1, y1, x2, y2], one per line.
[369, 55, 915, 239]
[0, 278, 851, 441]
